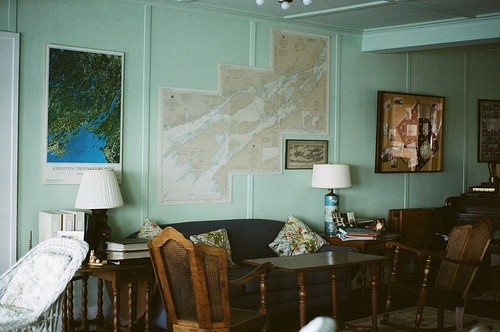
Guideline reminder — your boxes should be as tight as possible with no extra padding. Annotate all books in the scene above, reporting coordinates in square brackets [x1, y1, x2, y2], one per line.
[105, 239, 152, 265]
[39, 209, 84, 244]
[338, 227, 378, 242]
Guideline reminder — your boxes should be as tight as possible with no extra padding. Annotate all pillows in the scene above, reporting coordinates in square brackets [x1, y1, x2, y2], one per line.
[137, 218, 163, 240]
[268, 215, 328, 257]
[190, 228, 241, 269]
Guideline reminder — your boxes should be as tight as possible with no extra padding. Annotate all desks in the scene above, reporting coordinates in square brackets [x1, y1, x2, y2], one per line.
[316, 231, 402, 306]
[242, 248, 385, 332]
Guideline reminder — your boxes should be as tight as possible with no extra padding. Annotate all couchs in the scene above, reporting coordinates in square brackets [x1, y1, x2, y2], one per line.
[125, 217, 360, 322]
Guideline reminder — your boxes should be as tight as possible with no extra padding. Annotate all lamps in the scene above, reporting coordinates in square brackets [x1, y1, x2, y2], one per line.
[256, 0, 312, 10]
[75, 167, 124, 260]
[310, 163, 352, 235]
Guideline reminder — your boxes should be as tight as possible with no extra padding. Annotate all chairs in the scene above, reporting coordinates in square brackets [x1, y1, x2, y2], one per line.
[0, 234, 87, 332]
[379, 214, 496, 332]
[145, 227, 272, 331]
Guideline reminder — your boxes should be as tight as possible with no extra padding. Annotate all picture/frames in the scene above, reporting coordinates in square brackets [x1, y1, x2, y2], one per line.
[285, 138, 329, 169]
[374, 90, 446, 173]
[478, 98, 500, 164]
